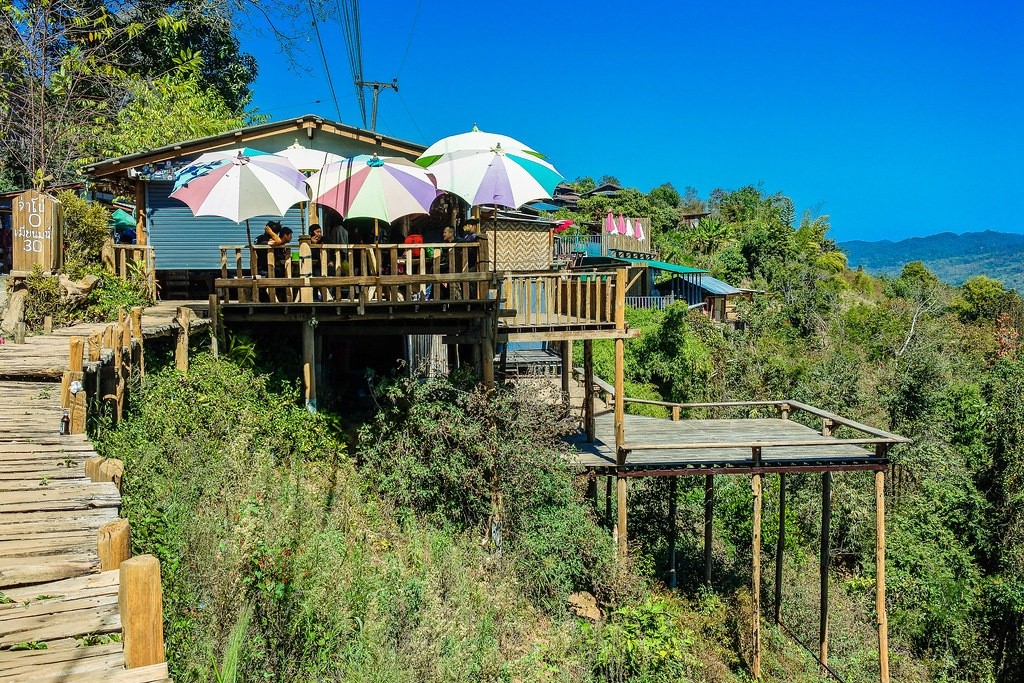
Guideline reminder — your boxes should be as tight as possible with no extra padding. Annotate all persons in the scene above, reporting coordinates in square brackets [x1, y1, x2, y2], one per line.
[303, 223, 349, 287]
[254, 220, 294, 278]
[442, 219, 478, 272]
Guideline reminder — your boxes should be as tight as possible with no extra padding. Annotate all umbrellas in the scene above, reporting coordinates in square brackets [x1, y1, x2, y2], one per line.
[302, 153, 449, 227]
[605, 208, 646, 243]
[415, 121, 566, 210]
[552, 219, 574, 233]
[168, 146, 310, 224]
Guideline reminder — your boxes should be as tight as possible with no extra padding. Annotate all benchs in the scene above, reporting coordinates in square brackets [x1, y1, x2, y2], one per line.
[234, 276, 265, 302]
[573, 367, 625, 409]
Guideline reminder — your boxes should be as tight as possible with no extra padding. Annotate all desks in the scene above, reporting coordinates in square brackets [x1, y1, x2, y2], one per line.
[397, 258, 431, 293]
[280, 259, 318, 302]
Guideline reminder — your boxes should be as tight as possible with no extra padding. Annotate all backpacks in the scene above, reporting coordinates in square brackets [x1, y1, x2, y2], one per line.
[405, 234, 424, 258]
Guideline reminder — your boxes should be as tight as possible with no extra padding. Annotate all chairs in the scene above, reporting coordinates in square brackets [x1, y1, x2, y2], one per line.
[366, 248, 405, 302]
